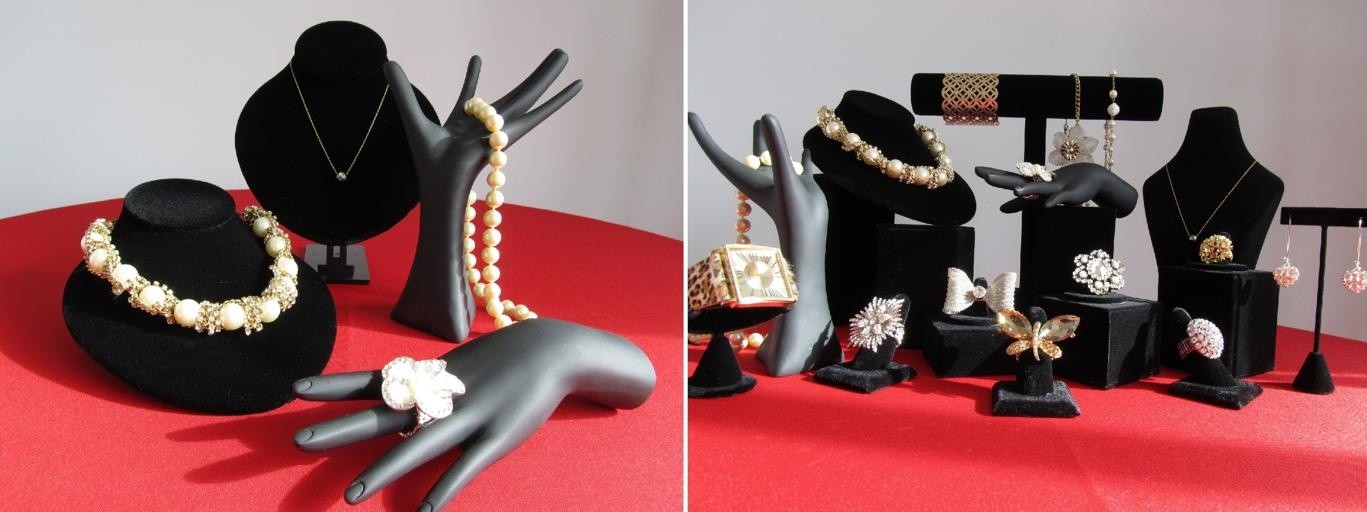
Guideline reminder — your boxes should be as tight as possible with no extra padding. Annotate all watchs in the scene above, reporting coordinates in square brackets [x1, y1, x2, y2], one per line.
[689, 240, 800, 314]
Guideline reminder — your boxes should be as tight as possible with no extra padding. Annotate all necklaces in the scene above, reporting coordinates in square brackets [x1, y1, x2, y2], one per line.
[1104, 68, 1121, 171]
[1048, 73, 1100, 167]
[816, 104, 954, 189]
[733, 147, 809, 350]
[75, 202, 299, 333]
[1166, 159, 1257, 241]
[284, 57, 393, 183]
[459, 92, 540, 336]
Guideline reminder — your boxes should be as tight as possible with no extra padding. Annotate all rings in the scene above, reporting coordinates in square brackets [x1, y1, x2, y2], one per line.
[1198, 233, 1234, 267]
[942, 265, 1016, 318]
[1177, 315, 1226, 362]
[849, 296, 907, 355]
[1015, 160, 1054, 200]
[383, 353, 466, 438]
[1072, 248, 1127, 296]
[998, 309, 1080, 362]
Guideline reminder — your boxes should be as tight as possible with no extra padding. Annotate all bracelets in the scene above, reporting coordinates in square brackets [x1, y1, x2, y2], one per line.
[942, 66, 1001, 126]
